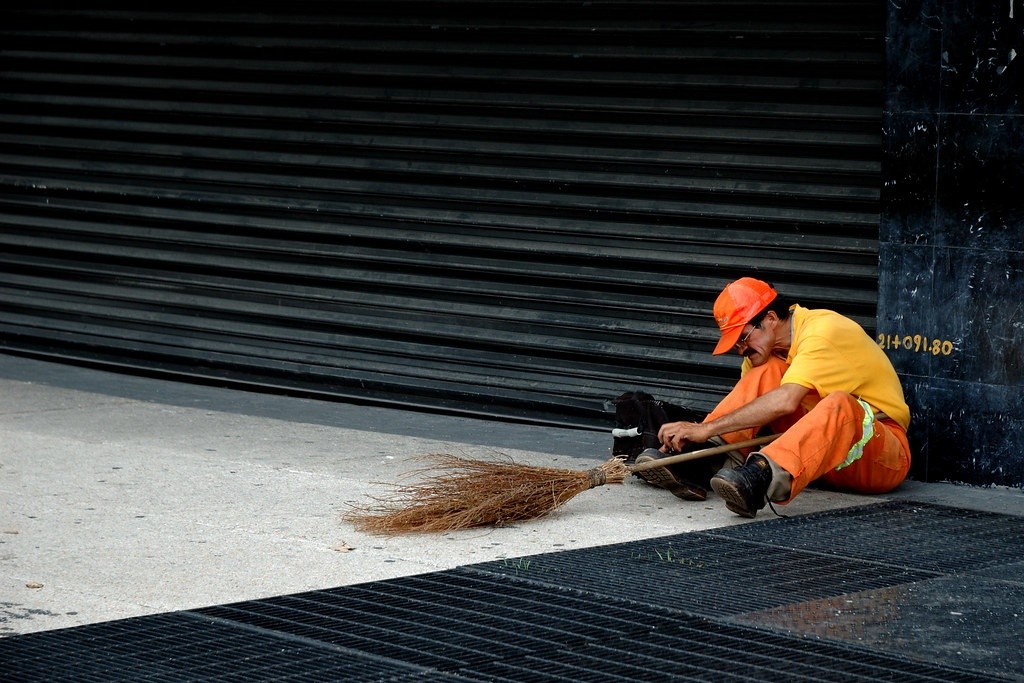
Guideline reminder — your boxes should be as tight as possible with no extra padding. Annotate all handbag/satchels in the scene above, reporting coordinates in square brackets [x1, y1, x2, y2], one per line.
[611, 390, 695, 463]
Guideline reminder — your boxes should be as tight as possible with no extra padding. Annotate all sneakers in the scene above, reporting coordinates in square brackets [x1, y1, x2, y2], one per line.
[635, 441, 712, 501]
[710, 452, 772, 519]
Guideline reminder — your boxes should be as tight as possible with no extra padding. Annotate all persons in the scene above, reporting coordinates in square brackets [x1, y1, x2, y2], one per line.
[635, 276, 912, 519]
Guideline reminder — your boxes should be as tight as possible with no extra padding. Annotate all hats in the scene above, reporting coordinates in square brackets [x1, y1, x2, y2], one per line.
[711, 277, 777, 356]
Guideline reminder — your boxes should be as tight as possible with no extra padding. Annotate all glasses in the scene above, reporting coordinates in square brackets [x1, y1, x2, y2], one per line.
[734, 320, 761, 350]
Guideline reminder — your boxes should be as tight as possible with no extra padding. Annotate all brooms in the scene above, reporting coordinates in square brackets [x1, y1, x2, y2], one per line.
[337, 410, 891, 539]
